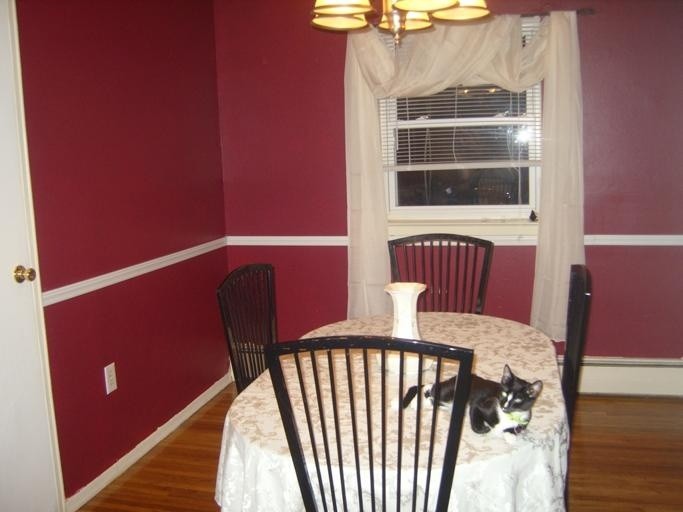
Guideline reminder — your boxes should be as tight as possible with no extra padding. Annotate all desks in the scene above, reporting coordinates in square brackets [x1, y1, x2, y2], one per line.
[214, 313, 572, 512]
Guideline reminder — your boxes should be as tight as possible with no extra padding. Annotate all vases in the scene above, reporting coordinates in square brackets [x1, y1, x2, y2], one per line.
[376, 282, 433, 374]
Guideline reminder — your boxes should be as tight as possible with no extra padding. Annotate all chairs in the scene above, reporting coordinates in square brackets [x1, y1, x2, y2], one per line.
[216, 263, 276, 394]
[561, 265, 591, 429]
[387, 232, 493, 313]
[264, 335, 475, 511]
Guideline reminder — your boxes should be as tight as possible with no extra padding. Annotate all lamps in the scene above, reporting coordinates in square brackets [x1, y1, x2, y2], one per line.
[308, 1, 490, 47]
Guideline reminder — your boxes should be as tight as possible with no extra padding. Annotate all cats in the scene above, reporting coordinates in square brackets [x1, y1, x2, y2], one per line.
[400, 361, 544, 438]
